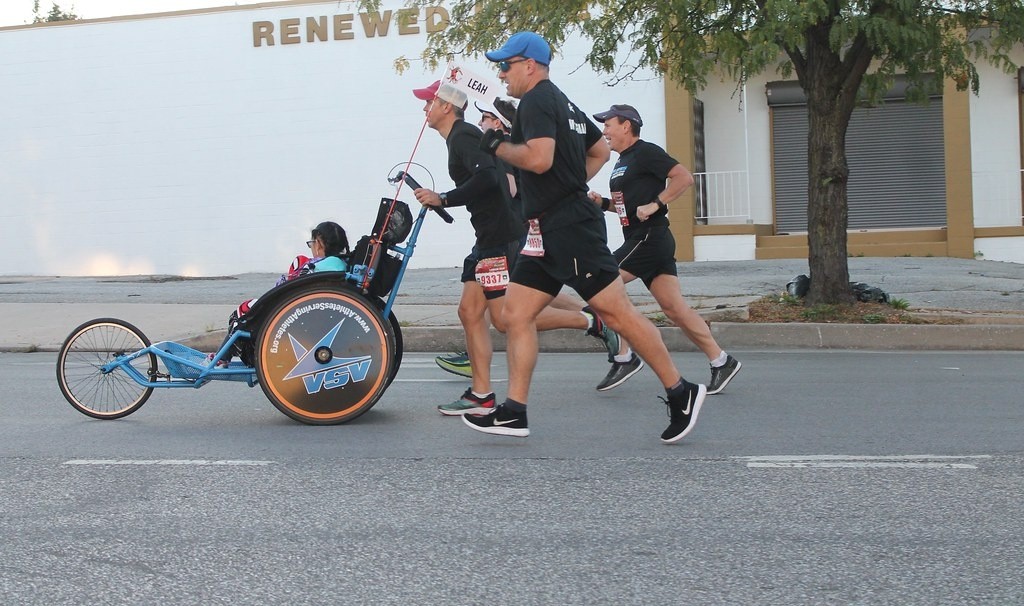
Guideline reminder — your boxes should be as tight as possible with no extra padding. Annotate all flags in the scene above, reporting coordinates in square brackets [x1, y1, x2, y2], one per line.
[442, 62, 500, 105]
[436, 85, 468, 108]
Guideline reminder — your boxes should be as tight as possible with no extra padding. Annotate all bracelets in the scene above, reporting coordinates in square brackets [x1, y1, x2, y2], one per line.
[653, 197, 663, 207]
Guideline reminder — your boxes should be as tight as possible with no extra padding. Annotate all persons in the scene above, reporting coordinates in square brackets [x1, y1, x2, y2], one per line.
[587, 103, 742, 394]
[435, 96, 585, 377]
[413, 79, 621, 414]
[201, 221, 350, 367]
[461, 31, 706, 442]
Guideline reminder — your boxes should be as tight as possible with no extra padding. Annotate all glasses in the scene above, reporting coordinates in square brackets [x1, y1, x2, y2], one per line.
[307, 240, 320, 248]
[481, 113, 494, 122]
[498, 58, 529, 72]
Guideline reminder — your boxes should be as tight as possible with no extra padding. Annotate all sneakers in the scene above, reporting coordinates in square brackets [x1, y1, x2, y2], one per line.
[199, 353, 230, 370]
[462, 403, 530, 437]
[582, 306, 621, 356]
[435, 348, 474, 378]
[657, 379, 707, 442]
[597, 353, 644, 391]
[438, 387, 496, 416]
[705, 354, 742, 394]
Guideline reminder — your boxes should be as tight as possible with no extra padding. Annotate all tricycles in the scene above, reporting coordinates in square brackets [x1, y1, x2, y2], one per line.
[54, 161, 455, 428]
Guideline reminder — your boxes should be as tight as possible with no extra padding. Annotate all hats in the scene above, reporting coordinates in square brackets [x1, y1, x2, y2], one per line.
[474, 99, 513, 129]
[593, 104, 643, 127]
[484, 32, 552, 65]
[413, 79, 468, 111]
